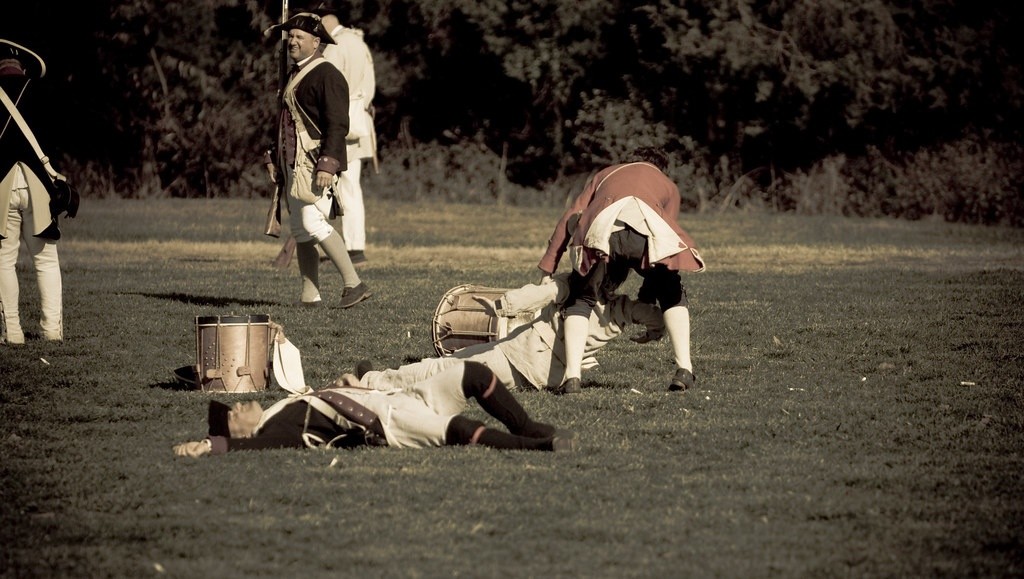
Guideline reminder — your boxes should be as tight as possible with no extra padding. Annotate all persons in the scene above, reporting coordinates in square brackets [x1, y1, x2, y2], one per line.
[314, 0, 377, 265]
[0, 39, 62, 347]
[171, 362, 586, 459]
[537, 162, 706, 398]
[355, 268, 666, 392]
[263, 12, 372, 309]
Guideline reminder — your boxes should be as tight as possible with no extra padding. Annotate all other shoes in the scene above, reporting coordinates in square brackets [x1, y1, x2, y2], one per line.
[668, 368, 694, 391]
[563, 377, 583, 394]
[337, 282, 372, 310]
[513, 420, 557, 440]
[320, 250, 366, 264]
[512, 439, 554, 453]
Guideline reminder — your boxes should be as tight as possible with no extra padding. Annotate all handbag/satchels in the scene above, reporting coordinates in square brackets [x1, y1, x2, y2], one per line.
[283, 121, 325, 204]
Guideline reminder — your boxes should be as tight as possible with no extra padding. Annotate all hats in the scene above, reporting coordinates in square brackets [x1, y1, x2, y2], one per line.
[275, 11, 337, 46]
[316, 0, 351, 28]
[208, 398, 231, 438]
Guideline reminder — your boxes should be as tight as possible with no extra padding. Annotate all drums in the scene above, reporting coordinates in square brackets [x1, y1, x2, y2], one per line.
[192, 313, 274, 395]
[429, 282, 543, 358]
